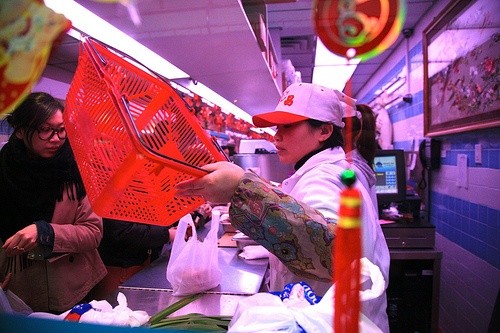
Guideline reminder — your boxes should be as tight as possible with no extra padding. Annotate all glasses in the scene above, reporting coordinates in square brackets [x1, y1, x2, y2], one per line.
[29, 126, 66, 141]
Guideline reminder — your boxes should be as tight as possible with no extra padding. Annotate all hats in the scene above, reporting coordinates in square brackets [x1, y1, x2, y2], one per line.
[332, 90, 361, 119]
[252, 82, 344, 128]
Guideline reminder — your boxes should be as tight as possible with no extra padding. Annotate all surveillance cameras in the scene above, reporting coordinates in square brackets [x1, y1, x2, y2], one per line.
[403, 94, 412, 103]
[402, 28, 413, 38]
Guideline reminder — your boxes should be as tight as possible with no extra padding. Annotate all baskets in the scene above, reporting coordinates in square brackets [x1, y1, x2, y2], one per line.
[62, 37, 232, 228]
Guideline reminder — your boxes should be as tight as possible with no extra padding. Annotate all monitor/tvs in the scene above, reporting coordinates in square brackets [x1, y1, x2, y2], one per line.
[373, 149, 406, 204]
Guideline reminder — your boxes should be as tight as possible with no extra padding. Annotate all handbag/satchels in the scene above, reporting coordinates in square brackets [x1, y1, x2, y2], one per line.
[167, 210, 222, 295]
[28, 291, 151, 327]
[228, 256, 386, 333]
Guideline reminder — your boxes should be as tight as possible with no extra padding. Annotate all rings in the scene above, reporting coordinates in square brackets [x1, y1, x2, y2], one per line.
[16, 246, 25, 252]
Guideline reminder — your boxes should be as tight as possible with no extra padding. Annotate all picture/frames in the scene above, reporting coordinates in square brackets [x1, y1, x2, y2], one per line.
[422, 0, 500, 135]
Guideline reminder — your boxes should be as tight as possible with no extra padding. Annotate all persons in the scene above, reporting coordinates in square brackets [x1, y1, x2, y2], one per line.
[96, 120, 237, 301]
[175, 83, 390, 333]
[0, 92, 116, 315]
